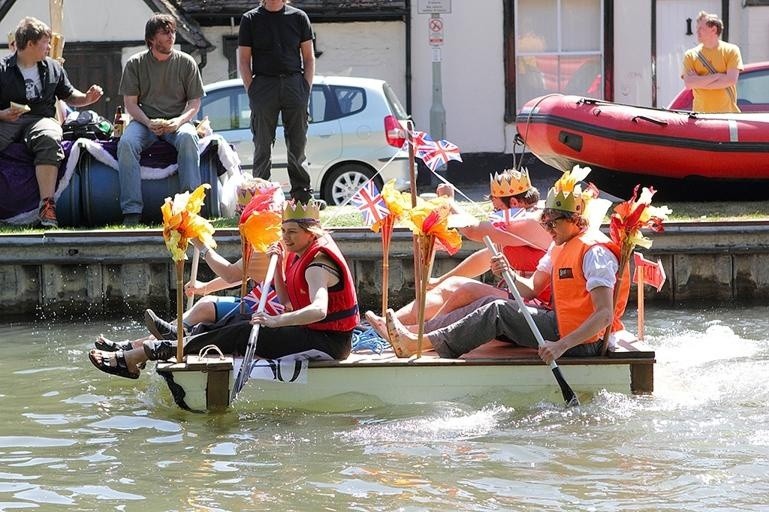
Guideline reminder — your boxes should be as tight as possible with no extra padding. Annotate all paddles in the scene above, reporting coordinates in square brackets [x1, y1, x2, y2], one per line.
[484, 233, 581, 408]
[228, 253, 278, 408]
[415, 156, 490, 218]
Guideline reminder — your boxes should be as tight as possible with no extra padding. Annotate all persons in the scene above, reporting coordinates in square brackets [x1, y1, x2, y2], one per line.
[89, 184, 284, 379]
[0, 18, 103, 228]
[117, 15, 207, 227]
[393, 164, 557, 325]
[142, 201, 361, 359]
[364, 186, 631, 364]
[238, 0, 327, 210]
[681, 11, 744, 113]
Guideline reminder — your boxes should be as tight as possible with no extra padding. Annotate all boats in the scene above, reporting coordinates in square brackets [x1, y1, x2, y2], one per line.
[515, 93, 769, 202]
[154, 325, 656, 418]
[520, 48, 602, 98]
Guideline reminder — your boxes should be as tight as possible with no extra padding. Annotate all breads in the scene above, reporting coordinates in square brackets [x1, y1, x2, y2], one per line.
[11, 102, 31, 114]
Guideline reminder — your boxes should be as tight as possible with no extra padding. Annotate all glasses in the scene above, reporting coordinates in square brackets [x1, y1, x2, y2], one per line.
[539, 215, 567, 229]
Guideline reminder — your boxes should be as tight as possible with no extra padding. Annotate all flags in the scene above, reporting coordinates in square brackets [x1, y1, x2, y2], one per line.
[419, 140, 463, 172]
[488, 208, 526, 231]
[353, 181, 390, 226]
[411, 132, 447, 171]
[243, 282, 285, 316]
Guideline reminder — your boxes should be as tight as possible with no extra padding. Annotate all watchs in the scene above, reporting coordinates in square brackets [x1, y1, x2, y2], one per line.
[199, 246, 210, 259]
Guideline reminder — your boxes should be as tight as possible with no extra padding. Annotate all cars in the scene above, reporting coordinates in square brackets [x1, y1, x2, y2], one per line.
[106, 75, 421, 213]
[667, 61, 769, 113]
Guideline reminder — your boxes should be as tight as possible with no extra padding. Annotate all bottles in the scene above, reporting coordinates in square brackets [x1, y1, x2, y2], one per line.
[113, 105, 124, 141]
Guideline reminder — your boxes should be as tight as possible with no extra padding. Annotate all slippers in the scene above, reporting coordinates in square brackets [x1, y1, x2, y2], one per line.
[94, 336, 145, 368]
[90, 349, 140, 378]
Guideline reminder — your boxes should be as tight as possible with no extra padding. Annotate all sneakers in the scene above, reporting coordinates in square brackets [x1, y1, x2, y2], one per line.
[384, 308, 410, 358]
[39, 197, 59, 228]
[365, 307, 392, 345]
[143, 307, 188, 341]
